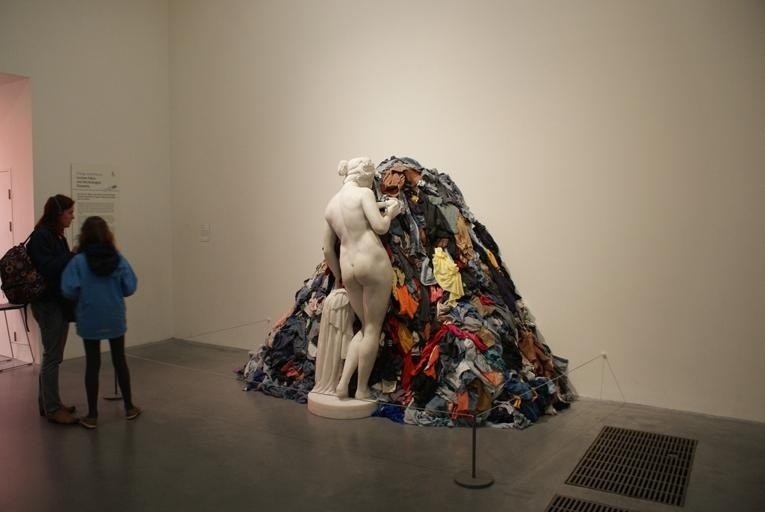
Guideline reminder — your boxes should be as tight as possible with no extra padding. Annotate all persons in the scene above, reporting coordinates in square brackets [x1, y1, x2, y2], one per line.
[20, 194, 79, 426]
[323, 156, 403, 400]
[60, 217, 143, 430]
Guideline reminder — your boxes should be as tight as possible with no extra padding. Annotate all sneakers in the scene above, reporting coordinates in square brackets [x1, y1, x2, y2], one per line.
[80, 414, 97, 429]
[43, 405, 80, 424]
[124, 406, 143, 419]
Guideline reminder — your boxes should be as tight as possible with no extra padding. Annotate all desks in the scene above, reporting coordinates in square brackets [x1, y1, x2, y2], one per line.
[0, 303, 36, 372]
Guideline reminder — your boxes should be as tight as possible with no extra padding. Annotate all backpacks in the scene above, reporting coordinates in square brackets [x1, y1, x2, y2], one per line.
[1, 231, 47, 305]
